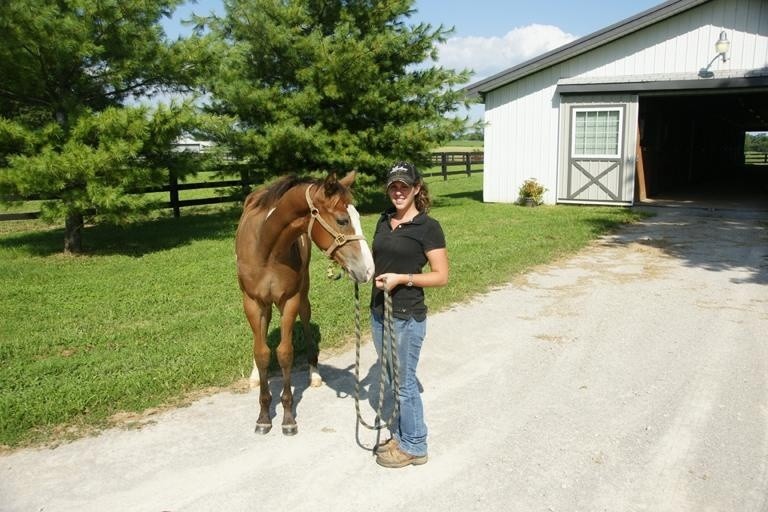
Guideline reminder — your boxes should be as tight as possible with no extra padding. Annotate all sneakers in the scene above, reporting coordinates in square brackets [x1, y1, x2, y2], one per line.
[375, 439, 427, 467]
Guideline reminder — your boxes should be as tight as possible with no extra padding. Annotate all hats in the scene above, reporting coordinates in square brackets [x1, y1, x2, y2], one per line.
[387, 161, 419, 189]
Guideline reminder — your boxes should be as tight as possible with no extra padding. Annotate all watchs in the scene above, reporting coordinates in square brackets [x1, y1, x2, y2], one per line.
[407, 273, 414, 288]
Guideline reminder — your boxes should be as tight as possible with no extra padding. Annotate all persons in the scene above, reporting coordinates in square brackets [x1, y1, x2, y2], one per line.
[370, 162, 449, 469]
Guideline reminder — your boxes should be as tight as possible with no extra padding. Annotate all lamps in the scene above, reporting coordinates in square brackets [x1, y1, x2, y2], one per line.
[716, 31, 729, 62]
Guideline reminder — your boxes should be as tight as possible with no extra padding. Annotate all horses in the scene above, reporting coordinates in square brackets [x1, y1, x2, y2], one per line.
[235, 165, 376, 436]
